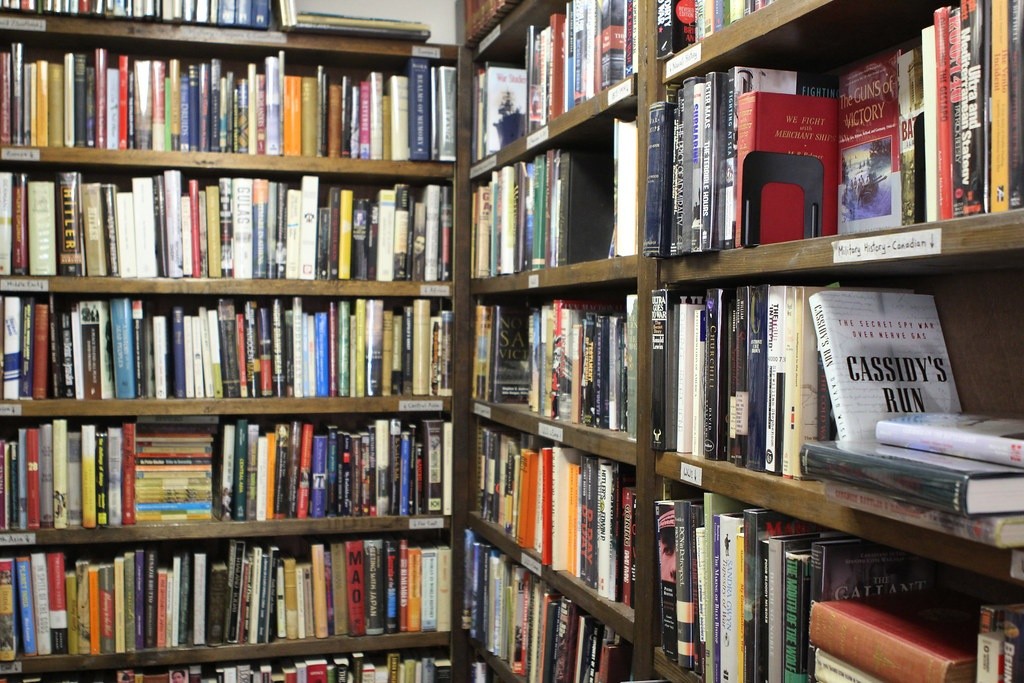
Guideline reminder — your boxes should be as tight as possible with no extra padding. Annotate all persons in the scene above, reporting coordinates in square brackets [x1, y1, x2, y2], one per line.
[171, 670, 186, 683]
[659, 502, 676, 583]
[412, 232, 426, 258]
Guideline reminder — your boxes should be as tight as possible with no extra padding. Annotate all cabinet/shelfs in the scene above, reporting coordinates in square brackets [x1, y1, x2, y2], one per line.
[0, 0, 1024, 683]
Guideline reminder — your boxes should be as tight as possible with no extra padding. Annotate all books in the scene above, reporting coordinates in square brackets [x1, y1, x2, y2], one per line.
[641, 0, 1023, 259]
[474, 420, 637, 614]
[1, 40, 458, 162]
[649, 283, 1023, 520]
[464, 0, 514, 47]
[0, 295, 454, 400]
[461, 527, 634, 683]
[469, 108, 642, 282]
[470, 0, 637, 163]
[0, 416, 451, 533]
[1, 537, 452, 664]
[470, 291, 638, 442]
[0, 0, 433, 43]
[0, 170, 453, 281]
[656, 476, 1024, 683]
[1, 651, 453, 683]
